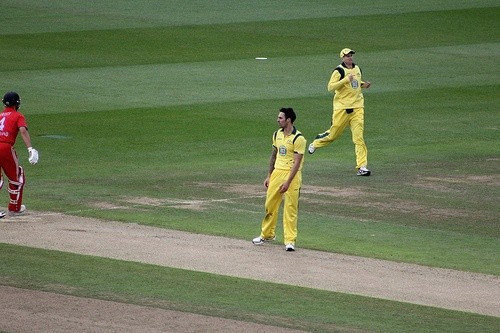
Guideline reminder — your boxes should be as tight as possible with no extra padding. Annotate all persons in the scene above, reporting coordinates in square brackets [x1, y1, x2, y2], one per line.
[0, 91, 39, 218]
[308, 47, 371, 177]
[253, 107, 307, 251]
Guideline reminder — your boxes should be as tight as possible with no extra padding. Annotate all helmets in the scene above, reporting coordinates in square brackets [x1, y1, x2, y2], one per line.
[1, 91, 20, 107]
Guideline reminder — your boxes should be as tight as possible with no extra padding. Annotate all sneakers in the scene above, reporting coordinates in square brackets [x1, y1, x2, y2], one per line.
[285, 244, 294, 251]
[357, 167, 370, 176]
[7, 204, 26, 216]
[0, 211, 7, 218]
[308, 143, 316, 154]
[252, 236, 276, 245]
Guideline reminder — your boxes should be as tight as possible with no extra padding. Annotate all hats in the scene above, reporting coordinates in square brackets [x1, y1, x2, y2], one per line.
[340, 47, 356, 59]
